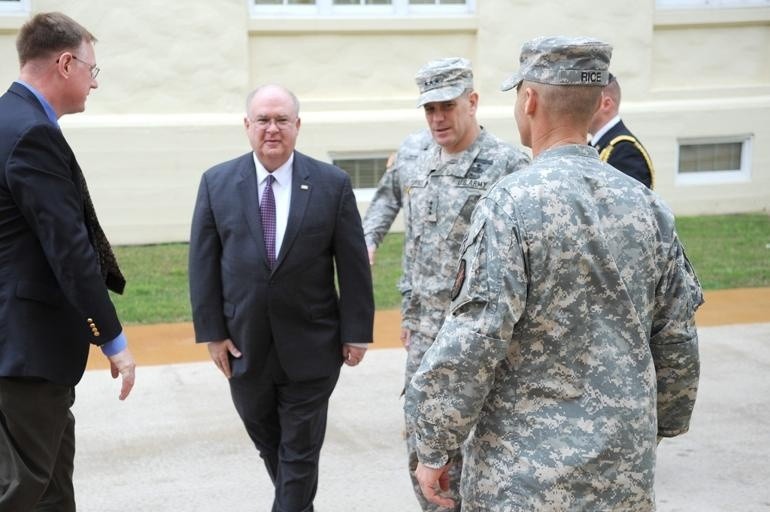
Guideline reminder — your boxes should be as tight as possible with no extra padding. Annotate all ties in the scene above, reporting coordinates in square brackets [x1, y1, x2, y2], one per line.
[260, 177, 277, 267]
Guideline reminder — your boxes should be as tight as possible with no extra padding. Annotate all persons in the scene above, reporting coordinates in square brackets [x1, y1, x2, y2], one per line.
[362, 60, 531, 512]
[404, 31, 703, 512]
[187, 84, 373, 512]
[588, 72, 654, 189]
[0, 13, 135, 512]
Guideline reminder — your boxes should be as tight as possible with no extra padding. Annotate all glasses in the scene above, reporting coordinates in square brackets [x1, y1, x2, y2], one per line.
[56, 53, 100, 81]
[248, 114, 298, 132]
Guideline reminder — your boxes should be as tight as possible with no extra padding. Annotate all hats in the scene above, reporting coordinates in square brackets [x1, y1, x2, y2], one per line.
[499, 33, 613, 91]
[414, 58, 475, 109]
